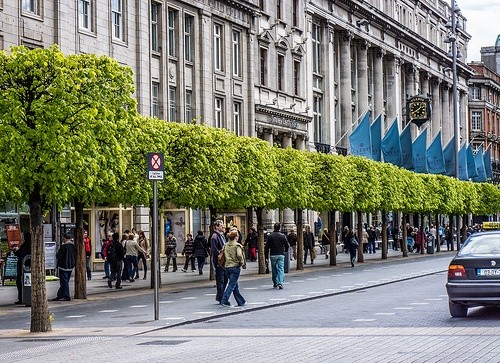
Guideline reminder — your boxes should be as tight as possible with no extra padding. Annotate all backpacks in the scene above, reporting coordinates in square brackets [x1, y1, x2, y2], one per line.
[106, 242, 118, 263]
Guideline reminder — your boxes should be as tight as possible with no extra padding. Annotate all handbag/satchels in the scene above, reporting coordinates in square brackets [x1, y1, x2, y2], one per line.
[351, 236, 359, 247]
[123, 242, 126, 255]
[311, 249, 317, 260]
[217, 245, 225, 265]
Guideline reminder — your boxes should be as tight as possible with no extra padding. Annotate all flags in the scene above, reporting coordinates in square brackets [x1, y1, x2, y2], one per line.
[348, 110, 493, 183]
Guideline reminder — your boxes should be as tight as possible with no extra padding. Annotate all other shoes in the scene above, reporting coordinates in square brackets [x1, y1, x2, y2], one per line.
[143, 278, 146, 280]
[88, 278, 91, 280]
[221, 303, 234, 308]
[107, 280, 112, 289]
[134, 276, 139, 279]
[172, 268, 177, 272]
[116, 286, 122, 289]
[164, 269, 168, 272]
[278, 284, 283, 289]
[103, 275, 109, 279]
[54, 296, 70, 301]
[14, 300, 22, 304]
[192, 270, 195, 272]
[351, 261, 354, 268]
[181, 268, 187, 272]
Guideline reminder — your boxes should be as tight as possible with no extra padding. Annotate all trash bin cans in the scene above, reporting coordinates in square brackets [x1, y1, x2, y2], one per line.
[427, 238, 434, 254]
[284, 242, 290, 273]
[22, 254, 31, 306]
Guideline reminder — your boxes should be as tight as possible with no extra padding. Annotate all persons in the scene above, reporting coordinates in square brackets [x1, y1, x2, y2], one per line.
[6, 220, 485, 306]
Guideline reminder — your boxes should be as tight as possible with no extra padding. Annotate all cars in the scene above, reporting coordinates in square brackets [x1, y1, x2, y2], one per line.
[445, 221, 500, 317]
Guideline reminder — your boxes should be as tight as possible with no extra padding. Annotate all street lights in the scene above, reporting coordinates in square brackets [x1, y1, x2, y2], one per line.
[444, 0, 463, 178]
